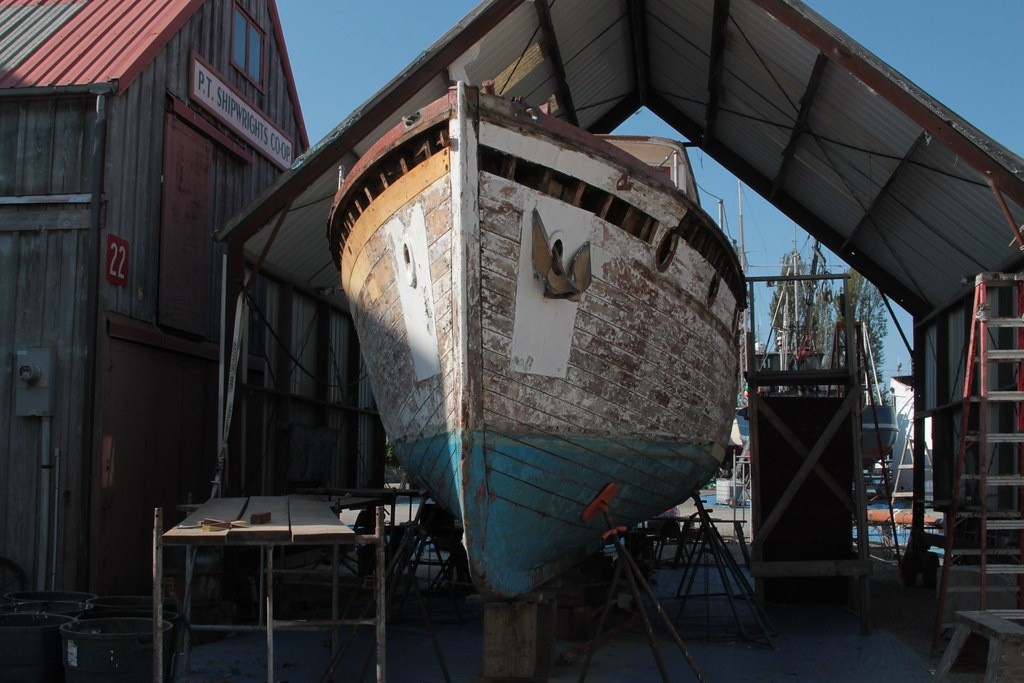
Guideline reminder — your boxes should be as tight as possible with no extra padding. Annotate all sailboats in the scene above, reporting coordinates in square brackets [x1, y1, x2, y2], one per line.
[736, 219, 899, 471]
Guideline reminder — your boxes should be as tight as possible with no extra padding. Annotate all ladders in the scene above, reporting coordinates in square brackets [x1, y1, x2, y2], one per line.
[930, 270, 1024, 662]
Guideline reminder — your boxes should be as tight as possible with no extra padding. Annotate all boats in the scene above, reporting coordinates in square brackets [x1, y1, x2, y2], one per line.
[329, 77, 749, 604]
